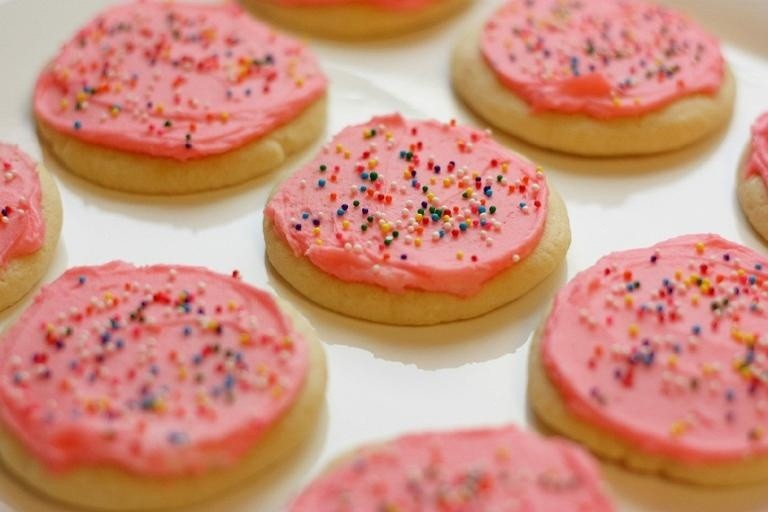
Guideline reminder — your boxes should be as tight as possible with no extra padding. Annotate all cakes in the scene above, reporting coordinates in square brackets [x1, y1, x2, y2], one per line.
[0, 0, 767, 512]
